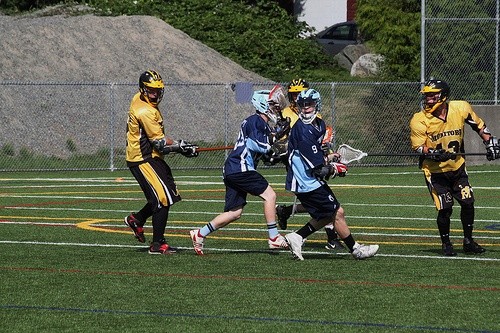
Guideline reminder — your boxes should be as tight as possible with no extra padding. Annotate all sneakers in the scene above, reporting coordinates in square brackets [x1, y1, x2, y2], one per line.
[285, 232, 306, 261]
[462, 238, 485, 253]
[190, 229, 205, 257]
[124, 213, 147, 243]
[149, 236, 177, 255]
[441, 241, 457, 256]
[275, 203, 291, 229]
[349, 240, 380, 259]
[325, 239, 346, 252]
[268, 234, 289, 249]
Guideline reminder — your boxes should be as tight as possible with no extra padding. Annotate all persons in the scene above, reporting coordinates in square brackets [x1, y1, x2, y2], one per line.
[410, 80, 499, 254]
[189, 80, 379, 261]
[123, 70, 198, 255]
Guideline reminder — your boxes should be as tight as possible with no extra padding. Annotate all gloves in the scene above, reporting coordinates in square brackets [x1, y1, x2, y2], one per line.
[273, 115, 291, 141]
[425, 147, 450, 163]
[324, 153, 341, 165]
[329, 162, 348, 179]
[483, 135, 500, 162]
[262, 145, 281, 166]
[177, 140, 199, 157]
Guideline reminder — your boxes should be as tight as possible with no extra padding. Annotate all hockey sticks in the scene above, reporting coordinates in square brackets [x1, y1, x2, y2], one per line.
[195, 146, 235, 153]
[336, 143, 491, 165]
[268, 83, 291, 137]
[322, 126, 344, 177]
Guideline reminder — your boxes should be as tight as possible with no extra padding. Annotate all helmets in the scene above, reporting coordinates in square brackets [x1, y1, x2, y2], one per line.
[419, 79, 450, 114]
[251, 90, 277, 124]
[287, 77, 309, 105]
[295, 88, 322, 124]
[139, 69, 165, 107]
